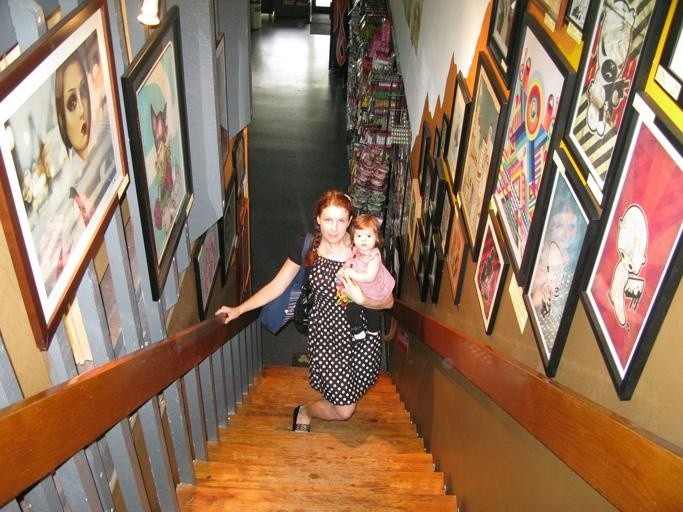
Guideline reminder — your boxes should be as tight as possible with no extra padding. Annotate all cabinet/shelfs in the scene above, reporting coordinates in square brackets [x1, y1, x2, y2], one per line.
[343, 0, 403, 214]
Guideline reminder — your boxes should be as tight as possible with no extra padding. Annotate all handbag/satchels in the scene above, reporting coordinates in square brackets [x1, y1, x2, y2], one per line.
[294, 292, 314, 335]
[258, 268, 305, 336]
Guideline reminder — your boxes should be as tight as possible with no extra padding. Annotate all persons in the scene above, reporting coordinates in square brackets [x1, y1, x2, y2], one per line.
[213, 189, 388, 431]
[52, 49, 93, 164]
[334, 211, 397, 343]
[525, 199, 581, 311]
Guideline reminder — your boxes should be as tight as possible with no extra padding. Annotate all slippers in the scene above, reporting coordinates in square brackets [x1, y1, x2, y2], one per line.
[293, 406, 310, 432]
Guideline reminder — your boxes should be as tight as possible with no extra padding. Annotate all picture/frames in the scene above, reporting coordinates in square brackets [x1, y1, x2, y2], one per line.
[0, 0, 130, 351]
[120, 5, 195, 301]
[194, 33, 252, 322]
[382, 0, 681, 401]
